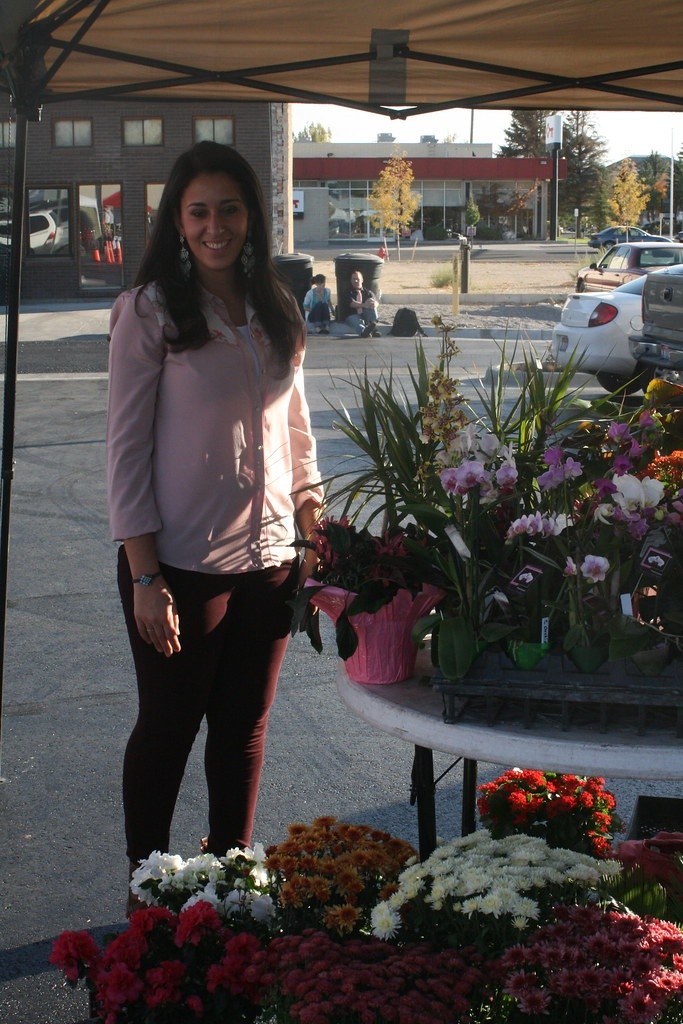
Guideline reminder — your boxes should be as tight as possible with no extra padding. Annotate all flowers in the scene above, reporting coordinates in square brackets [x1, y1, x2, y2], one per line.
[291, 316, 683, 688]
[46, 767, 683, 1024]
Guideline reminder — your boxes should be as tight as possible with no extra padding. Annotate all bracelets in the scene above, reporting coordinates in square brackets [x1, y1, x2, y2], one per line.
[133, 572, 162, 585]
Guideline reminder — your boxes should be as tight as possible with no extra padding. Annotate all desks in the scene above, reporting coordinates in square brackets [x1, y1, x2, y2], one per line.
[336, 633, 683, 861]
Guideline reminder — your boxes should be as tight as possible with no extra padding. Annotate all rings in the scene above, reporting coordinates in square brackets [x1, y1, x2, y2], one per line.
[147, 629, 154, 632]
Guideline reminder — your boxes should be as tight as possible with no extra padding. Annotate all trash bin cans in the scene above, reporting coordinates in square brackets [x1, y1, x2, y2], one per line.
[273, 253, 315, 320]
[333, 252, 383, 322]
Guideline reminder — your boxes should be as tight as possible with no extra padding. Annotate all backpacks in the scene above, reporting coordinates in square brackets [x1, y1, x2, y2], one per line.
[391, 307, 426, 338]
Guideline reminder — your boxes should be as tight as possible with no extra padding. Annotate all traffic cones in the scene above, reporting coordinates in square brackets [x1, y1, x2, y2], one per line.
[93, 240, 122, 265]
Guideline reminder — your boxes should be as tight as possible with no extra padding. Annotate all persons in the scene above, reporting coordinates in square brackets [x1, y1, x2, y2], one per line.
[303, 274, 335, 334]
[343, 271, 382, 337]
[105, 139, 332, 907]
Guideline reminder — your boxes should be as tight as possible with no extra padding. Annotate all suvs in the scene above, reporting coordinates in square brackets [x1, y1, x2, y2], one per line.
[0, 205, 68, 255]
[627, 264, 683, 371]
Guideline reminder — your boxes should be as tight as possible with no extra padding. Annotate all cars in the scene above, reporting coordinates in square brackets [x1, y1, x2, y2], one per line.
[574, 241, 683, 293]
[551, 274, 648, 395]
[588, 226, 674, 251]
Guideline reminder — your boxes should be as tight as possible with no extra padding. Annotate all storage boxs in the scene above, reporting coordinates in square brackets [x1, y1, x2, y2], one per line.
[623, 794, 683, 842]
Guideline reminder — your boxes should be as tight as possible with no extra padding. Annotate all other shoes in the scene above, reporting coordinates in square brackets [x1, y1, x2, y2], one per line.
[316, 327, 330, 334]
[372, 329, 381, 337]
[363, 321, 375, 337]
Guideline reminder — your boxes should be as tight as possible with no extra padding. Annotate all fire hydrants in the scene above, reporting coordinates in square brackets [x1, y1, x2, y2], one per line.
[377, 247, 386, 261]
